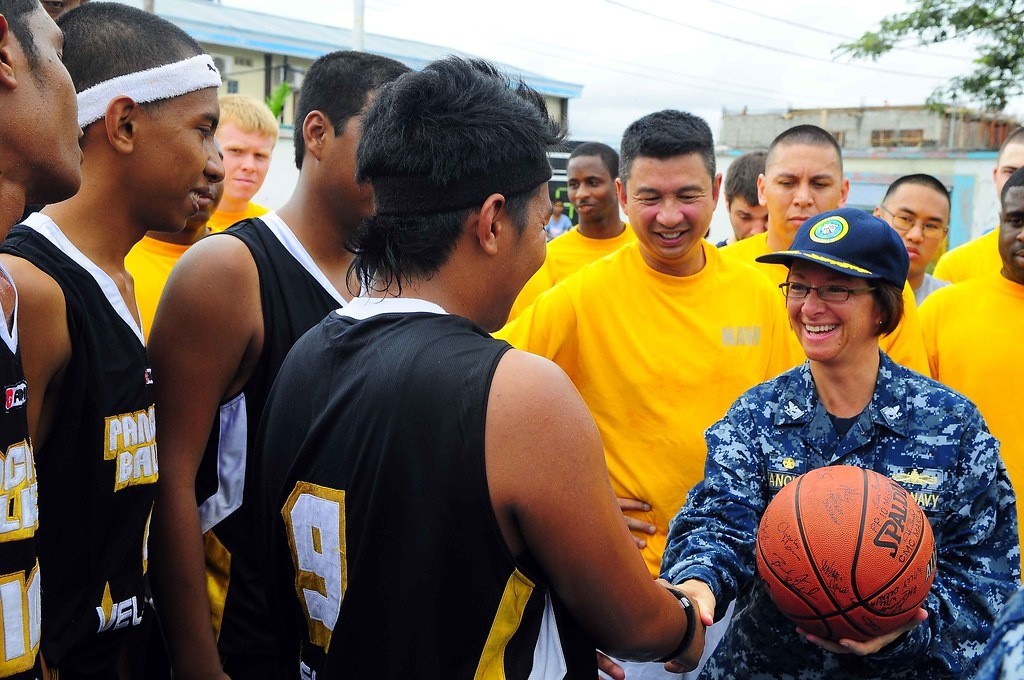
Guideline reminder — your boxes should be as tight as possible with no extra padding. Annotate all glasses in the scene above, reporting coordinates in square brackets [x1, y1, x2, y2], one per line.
[779, 282, 876, 302]
[882, 207, 948, 240]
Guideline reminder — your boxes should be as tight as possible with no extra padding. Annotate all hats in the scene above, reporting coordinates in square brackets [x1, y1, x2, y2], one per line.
[755, 208, 910, 290]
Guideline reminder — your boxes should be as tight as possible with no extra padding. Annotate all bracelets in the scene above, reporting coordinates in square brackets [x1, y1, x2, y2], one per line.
[660, 588, 696, 662]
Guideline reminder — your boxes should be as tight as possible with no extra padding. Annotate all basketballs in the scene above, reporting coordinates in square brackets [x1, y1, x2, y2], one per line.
[753, 463, 940, 644]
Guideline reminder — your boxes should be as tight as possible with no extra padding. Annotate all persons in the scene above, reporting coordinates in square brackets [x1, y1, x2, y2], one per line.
[496, 109, 809, 679]
[714, 150, 767, 247]
[149, 51, 412, 680]
[918, 166, 1023, 582]
[0, 0, 277, 680]
[713, 125, 930, 377]
[545, 199, 571, 243]
[656, 207, 1024, 680]
[934, 125, 1024, 286]
[505, 144, 636, 327]
[875, 174, 954, 306]
[216, 57, 707, 679]
